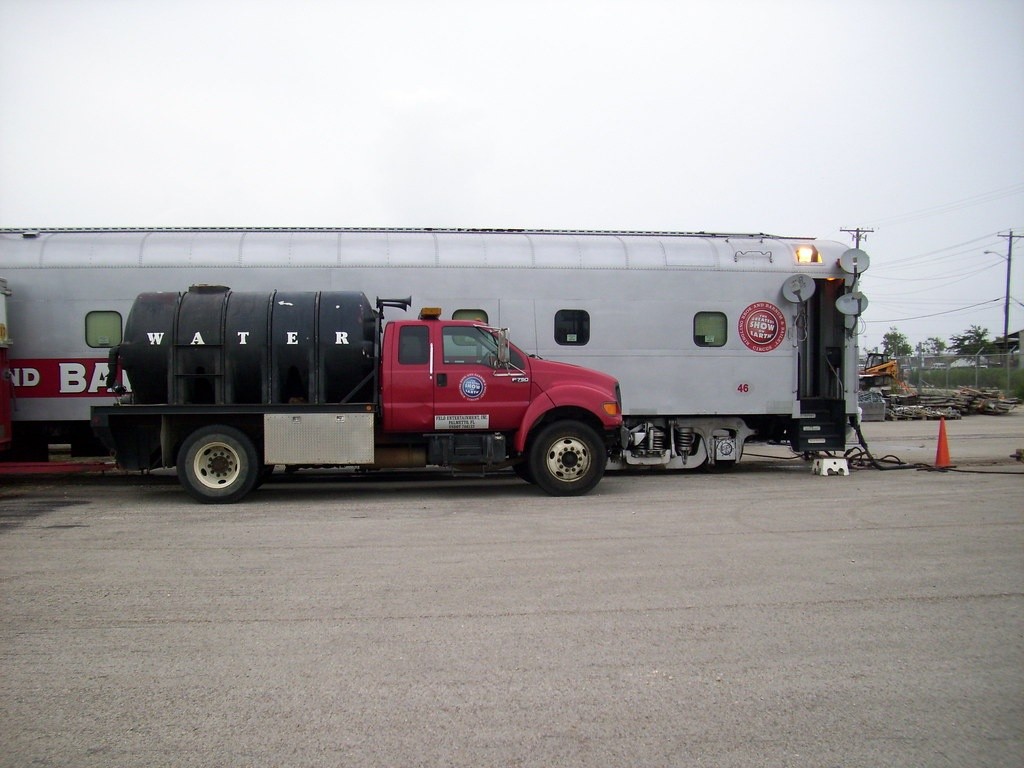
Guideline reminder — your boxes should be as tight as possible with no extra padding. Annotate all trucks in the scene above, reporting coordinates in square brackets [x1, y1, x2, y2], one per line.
[0, 225, 869, 470]
[95, 279, 626, 504]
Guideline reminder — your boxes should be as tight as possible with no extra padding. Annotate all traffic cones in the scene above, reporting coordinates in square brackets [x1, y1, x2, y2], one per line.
[935, 414, 956, 473]
